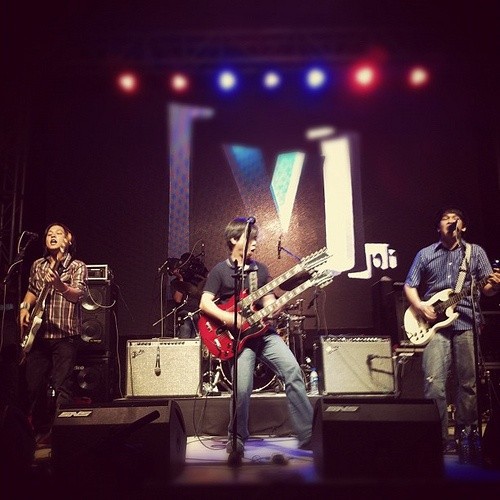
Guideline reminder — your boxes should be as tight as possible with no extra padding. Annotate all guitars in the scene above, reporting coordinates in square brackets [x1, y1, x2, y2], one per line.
[402, 268, 500, 346]
[196, 244, 337, 360]
[19, 231, 75, 353]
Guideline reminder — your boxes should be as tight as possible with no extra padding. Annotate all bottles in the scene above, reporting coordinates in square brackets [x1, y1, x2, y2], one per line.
[461, 429, 469, 456]
[473, 430, 482, 456]
[310, 368, 318, 395]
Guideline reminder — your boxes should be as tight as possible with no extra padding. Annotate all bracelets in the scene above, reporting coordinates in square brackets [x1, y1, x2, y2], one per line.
[20, 301, 30, 311]
[62, 286, 70, 295]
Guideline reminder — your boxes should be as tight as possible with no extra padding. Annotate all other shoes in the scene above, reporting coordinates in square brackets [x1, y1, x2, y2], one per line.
[297, 438, 312, 450]
[33, 432, 53, 459]
[226, 434, 246, 453]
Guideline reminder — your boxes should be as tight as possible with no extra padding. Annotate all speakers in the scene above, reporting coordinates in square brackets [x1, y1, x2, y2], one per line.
[317, 335, 397, 395]
[311, 398, 443, 475]
[52, 399, 187, 474]
[69, 280, 118, 403]
[127, 338, 202, 397]
[399, 356, 425, 400]
[0, 404, 34, 469]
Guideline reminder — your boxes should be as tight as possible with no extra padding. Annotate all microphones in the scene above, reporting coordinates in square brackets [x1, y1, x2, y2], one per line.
[368, 354, 378, 361]
[24, 231, 38, 238]
[449, 218, 460, 232]
[278, 237, 282, 259]
[247, 216, 256, 224]
[154, 347, 161, 376]
[201, 241, 205, 256]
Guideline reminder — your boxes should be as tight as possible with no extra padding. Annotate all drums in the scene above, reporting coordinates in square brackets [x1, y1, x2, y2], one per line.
[214, 359, 277, 393]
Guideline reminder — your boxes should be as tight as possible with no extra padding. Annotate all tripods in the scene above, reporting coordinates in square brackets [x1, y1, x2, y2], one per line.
[187, 223, 288, 480]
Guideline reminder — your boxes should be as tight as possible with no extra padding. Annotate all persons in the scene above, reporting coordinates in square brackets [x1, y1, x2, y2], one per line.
[404, 207, 500, 460]
[16, 223, 89, 462]
[170, 252, 195, 339]
[198, 217, 316, 453]
